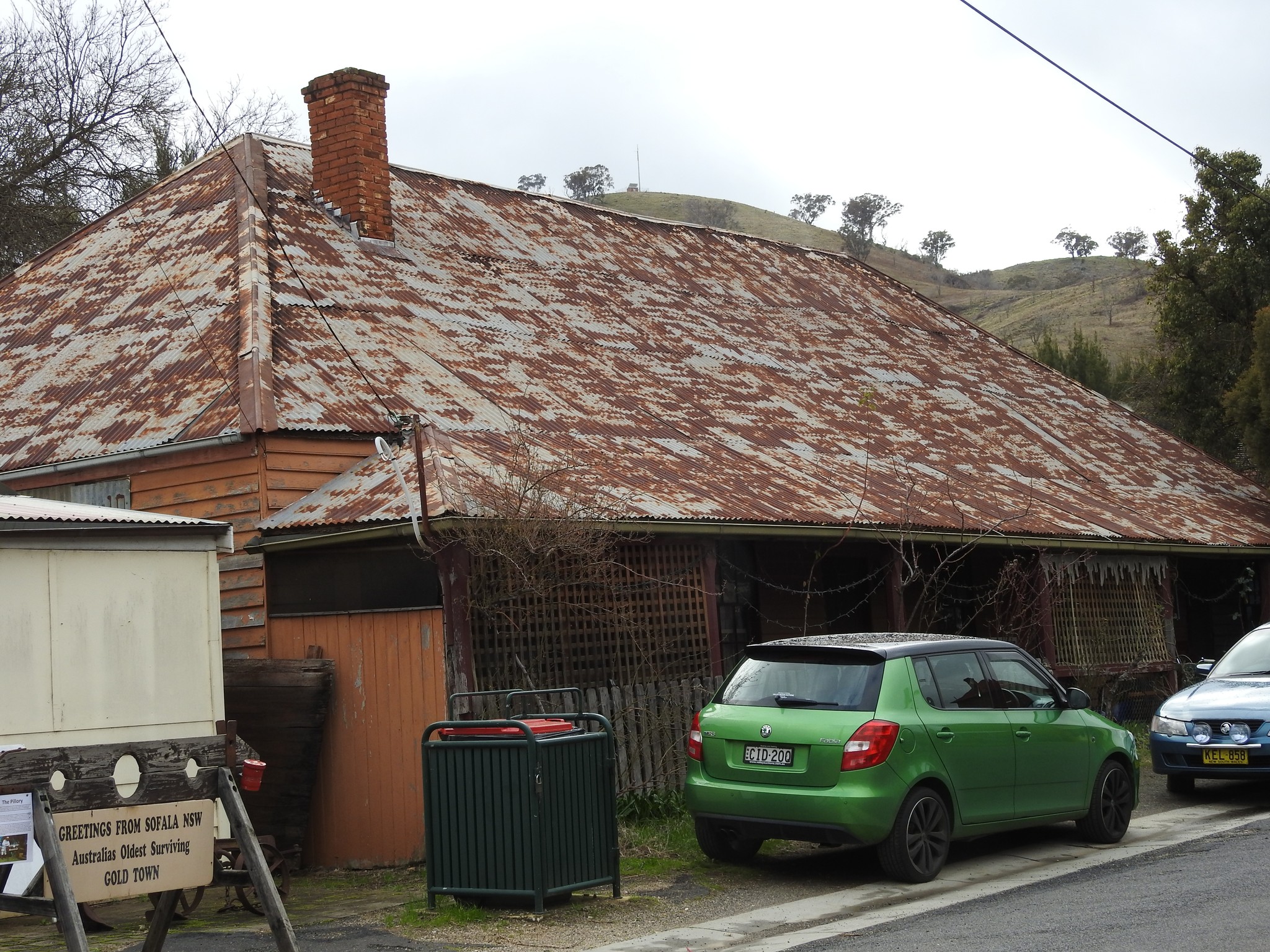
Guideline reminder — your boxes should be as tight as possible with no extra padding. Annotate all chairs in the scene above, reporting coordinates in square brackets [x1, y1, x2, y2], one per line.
[930, 658, 971, 707]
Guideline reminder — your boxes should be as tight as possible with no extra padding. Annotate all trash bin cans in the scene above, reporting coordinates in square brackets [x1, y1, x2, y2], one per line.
[421, 712, 620, 912]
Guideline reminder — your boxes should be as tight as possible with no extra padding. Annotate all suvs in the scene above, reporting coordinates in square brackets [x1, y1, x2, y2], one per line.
[682, 630, 1141, 884]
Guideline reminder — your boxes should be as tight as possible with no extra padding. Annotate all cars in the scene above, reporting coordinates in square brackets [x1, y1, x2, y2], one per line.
[1148, 618, 1270, 795]
